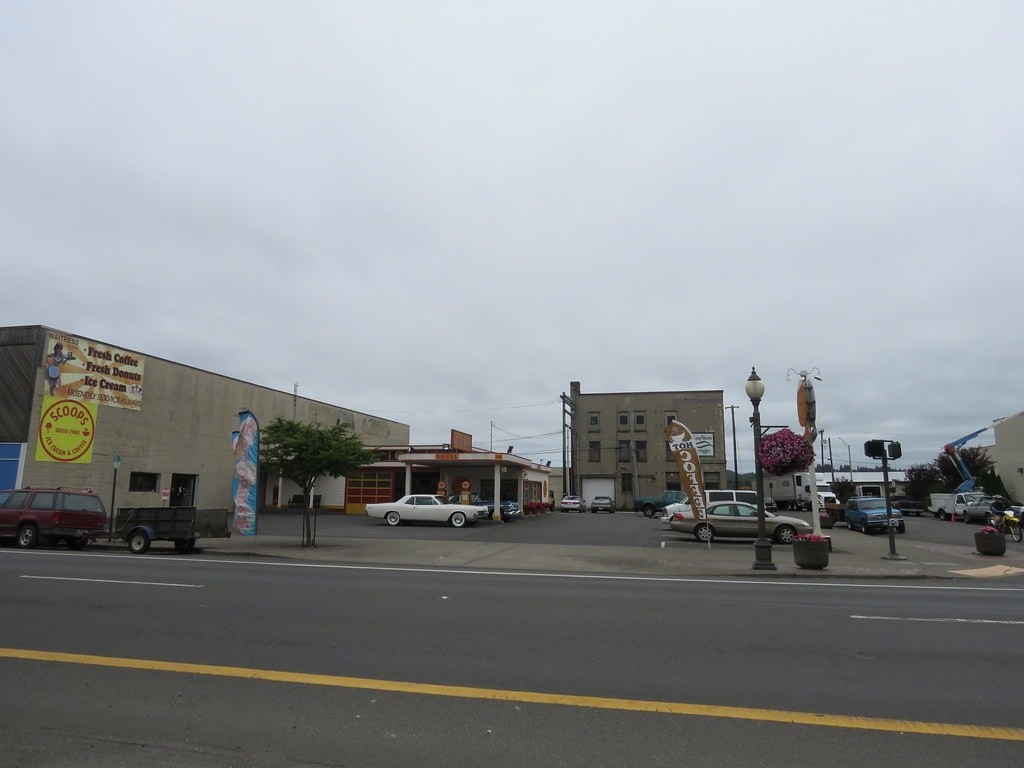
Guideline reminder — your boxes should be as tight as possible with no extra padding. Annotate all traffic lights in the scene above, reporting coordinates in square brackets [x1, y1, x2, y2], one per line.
[863, 441, 884, 458]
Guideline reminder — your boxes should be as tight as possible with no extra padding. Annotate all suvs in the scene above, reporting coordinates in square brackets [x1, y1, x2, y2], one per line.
[844, 495, 907, 535]
[0, 485, 107, 550]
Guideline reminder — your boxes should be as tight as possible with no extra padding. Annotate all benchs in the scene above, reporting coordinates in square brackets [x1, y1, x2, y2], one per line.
[288, 494, 321, 510]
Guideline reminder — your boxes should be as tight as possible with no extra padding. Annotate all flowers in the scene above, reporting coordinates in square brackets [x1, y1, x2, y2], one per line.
[525, 502, 551, 508]
[794, 534, 828, 544]
[759, 428, 816, 478]
[819, 512, 832, 519]
[980, 525, 1000, 536]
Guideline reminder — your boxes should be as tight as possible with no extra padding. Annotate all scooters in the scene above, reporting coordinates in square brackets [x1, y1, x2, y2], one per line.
[986, 501, 1022, 542]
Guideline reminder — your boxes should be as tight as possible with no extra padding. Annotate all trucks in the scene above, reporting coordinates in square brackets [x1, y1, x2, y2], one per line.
[750, 472, 841, 512]
[926, 490, 990, 522]
[890, 495, 926, 517]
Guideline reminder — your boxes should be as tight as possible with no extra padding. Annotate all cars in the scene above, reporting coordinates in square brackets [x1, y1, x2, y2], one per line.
[668, 500, 814, 545]
[591, 495, 616, 513]
[446, 492, 521, 521]
[764, 496, 777, 512]
[559, 495, 587, 513]
[364, 493, 490, 529]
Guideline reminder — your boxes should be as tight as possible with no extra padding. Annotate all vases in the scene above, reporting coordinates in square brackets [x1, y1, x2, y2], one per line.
[770, 458, 798, 477]
[524, 506, 531, 514]
[540, 505, 546, 514]
[975, 532, 1008, 555]
[795, 541, 829, 569]
[532, 507, 538, 514]
[820, 518, 834, 529]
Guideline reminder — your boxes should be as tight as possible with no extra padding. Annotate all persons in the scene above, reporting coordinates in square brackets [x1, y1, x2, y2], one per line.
[990, 495, 1011, 528]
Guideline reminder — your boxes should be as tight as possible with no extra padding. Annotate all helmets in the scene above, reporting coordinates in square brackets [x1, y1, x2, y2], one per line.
[993, 494, 1003, 499]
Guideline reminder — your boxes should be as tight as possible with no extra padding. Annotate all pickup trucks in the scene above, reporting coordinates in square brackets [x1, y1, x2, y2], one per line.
[963, 494, 1024, 524]
[632, 490, 687, 517]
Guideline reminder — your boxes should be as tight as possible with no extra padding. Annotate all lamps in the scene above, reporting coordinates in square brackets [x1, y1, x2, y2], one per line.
[1016, 468, 1023, 473]
[442, 444, 450, 452]
[507, 445, 513, 454]
[408, 446, 415, 453]
[546, 461, 551, 467]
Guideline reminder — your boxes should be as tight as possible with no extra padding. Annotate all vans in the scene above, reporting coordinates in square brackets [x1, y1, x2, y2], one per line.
[661, 489, 766, 523]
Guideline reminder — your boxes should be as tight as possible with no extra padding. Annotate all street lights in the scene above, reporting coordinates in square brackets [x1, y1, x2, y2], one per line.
[744, 366, 778, 571]
[838, 437, 853, 486]
[783, 365, 833, 552]
